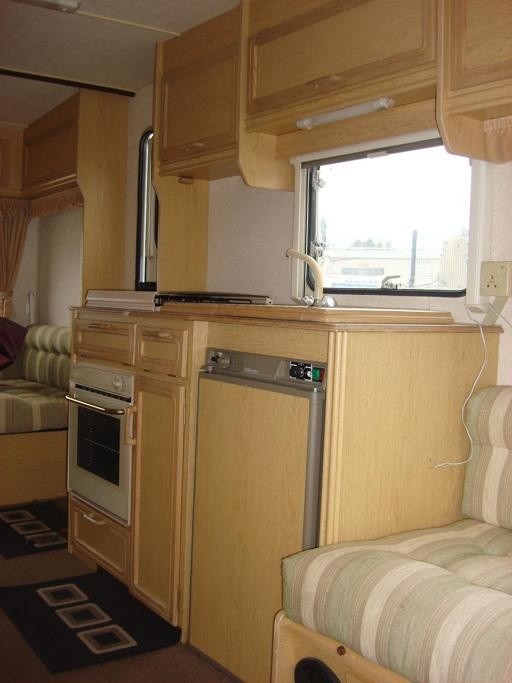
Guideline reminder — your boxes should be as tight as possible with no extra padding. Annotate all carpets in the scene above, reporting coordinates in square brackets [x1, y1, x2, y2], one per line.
[1, 498, 182, 673]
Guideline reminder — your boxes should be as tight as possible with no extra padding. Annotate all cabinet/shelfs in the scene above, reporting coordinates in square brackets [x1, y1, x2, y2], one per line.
[0, 91, 78, 200]
[159, 0, 511, 186]
[68, 308, 504, 682]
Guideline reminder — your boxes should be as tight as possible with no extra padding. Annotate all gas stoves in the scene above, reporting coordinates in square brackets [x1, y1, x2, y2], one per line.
[87, 290, 270, 304]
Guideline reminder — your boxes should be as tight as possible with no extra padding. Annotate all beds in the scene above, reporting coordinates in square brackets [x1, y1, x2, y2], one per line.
[1, 323, 72, 508]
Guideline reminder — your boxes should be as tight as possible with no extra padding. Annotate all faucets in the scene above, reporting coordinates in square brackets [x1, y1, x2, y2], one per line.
[285, 248, 322, 301]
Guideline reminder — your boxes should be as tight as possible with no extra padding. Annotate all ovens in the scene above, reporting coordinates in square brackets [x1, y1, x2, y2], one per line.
[66, 364, 137, 524]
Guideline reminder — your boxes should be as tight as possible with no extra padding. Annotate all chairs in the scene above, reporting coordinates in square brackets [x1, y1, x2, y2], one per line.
[273, 384, 512, 683]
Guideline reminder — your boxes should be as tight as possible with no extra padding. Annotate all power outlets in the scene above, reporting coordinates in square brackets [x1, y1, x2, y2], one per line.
[482, 261, 512, 295]
